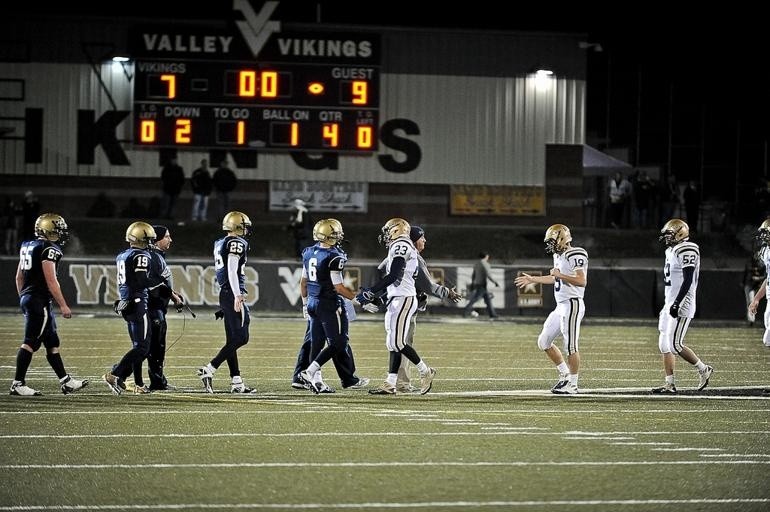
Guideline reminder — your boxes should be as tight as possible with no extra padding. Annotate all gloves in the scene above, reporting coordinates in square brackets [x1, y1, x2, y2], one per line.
[670, 305, 679, 318]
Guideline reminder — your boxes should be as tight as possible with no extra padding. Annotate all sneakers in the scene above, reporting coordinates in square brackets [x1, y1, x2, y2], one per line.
[698, 365, 713, 391]
[197, 369, 257, 394]
[551, 381, 578, 394]
[654, 384, 676, 394]
[10, 384, 41, 395]
[349, 368, 435, 394]
[102, 370, 176, 394]
[292, 371, 335, 393]
[60, 377, 88, 393]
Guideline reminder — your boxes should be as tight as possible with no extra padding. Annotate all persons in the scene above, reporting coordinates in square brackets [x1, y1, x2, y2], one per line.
[463, 252, 499, 318]
[288, 199, 462, 396]
[514, 224, 589, 395]
[604, 167, 704, 232]
[86, 156, 239, 223]
[111, 225, 184, 392]
[749, 219, 770, 348]
[2, 190, 41, 255]
[10, 213, 89, 396]
[102, 222, 171, 396]
[196, 211, 258, 393]
[651, 219, 714, 395]
[742, 250, 767, 326]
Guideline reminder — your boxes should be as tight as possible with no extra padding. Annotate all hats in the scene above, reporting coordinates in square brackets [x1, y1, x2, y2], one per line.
[410, 226, 424, 243]
[152, 226, 167, 243]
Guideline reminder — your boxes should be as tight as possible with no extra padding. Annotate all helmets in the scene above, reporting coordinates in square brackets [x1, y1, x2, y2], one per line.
[223, 211, 252, 236]
[313, 219, 343, 245]
[660, 219, 690, 245]
[382, 218, 411, 240]
[125, 222, 157, 243]
[757, 219, 770, 232]
[35, 214, 66, 241]
[544, 224, 572, 248]
